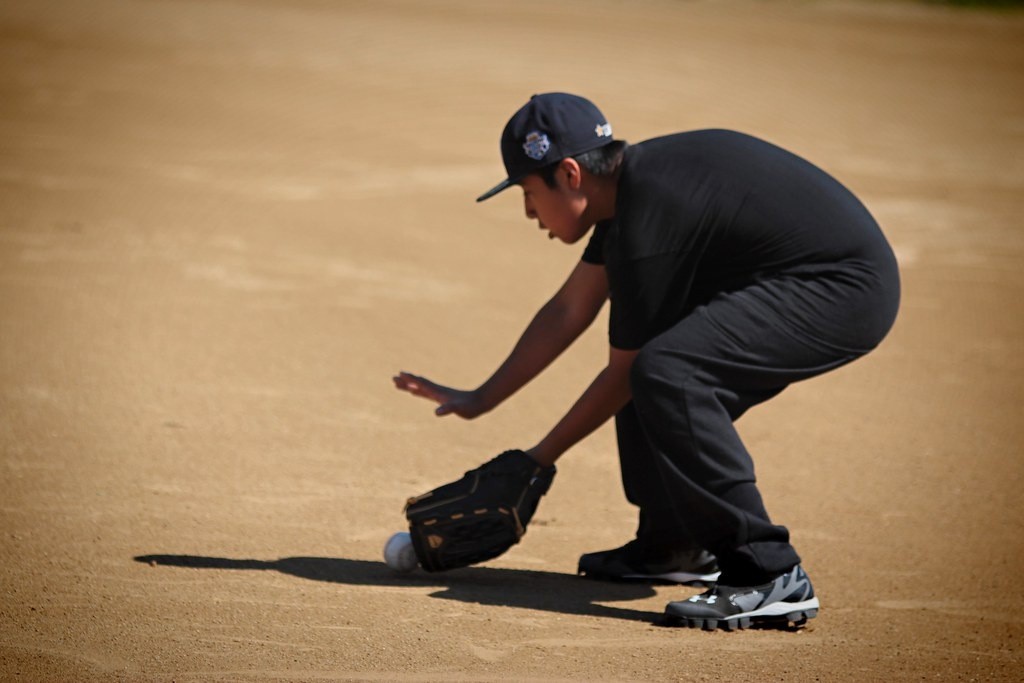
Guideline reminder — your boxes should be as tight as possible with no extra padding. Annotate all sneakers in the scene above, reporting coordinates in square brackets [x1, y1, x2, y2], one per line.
[665, 564, 820, 632]
[578, 537, 721, 587]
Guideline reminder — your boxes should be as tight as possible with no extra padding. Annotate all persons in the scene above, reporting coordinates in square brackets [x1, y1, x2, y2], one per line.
[392, 93, 901, 632]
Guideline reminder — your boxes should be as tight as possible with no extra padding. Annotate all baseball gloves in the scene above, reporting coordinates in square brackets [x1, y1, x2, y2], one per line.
[402, 448, 557, 577]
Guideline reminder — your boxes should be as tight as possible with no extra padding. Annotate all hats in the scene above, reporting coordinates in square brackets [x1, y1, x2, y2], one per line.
[476, 92, 613, 202]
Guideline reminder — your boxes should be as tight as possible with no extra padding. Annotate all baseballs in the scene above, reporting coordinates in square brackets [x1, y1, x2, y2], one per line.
[383, 532, 423, 573]
